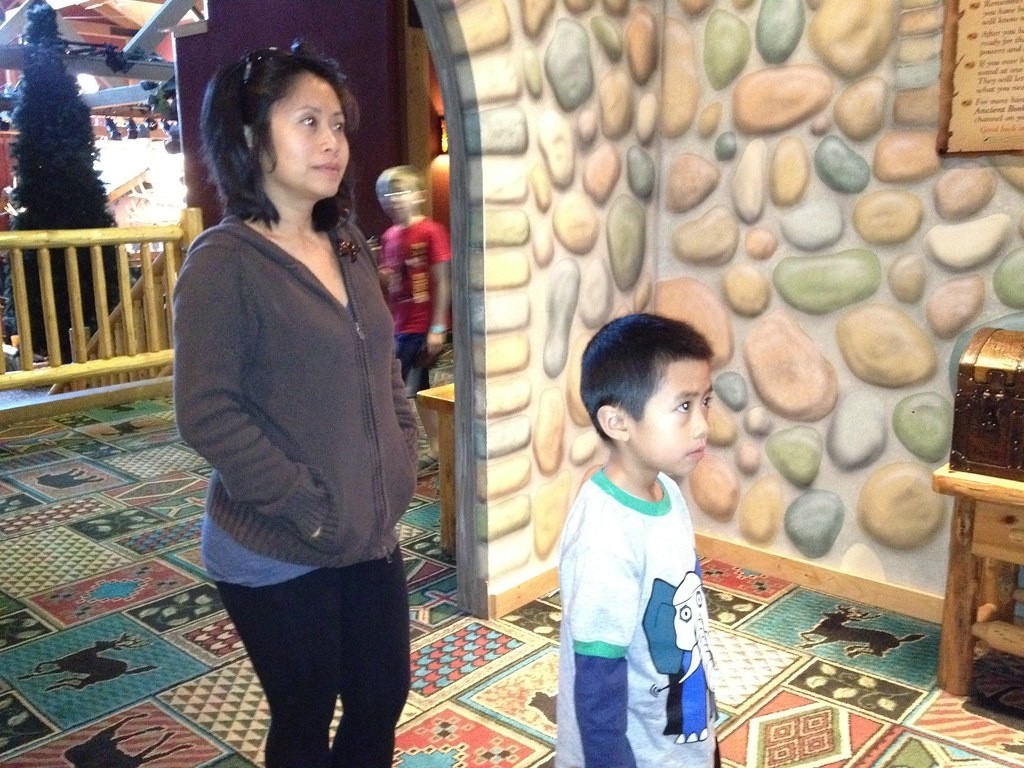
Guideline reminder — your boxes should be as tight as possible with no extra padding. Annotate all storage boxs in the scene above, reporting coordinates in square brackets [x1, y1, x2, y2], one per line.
[949, 327, 1024, 480]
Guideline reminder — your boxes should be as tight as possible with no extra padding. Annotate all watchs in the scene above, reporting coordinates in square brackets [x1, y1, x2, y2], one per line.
[427, 324, 448, 335]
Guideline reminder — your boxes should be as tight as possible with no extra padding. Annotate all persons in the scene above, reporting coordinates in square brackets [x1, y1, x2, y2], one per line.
[172, 42, 419, 768]
[376, 165, 451, 475]
[554, 313, 722, 768]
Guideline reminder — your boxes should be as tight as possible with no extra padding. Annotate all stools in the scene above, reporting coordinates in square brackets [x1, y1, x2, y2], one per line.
[416, 381, 454, 554]
[932, 462, 1024, 696]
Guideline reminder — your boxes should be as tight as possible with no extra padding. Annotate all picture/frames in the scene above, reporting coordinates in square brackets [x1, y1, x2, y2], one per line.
[935, 0, 1023, 158]
